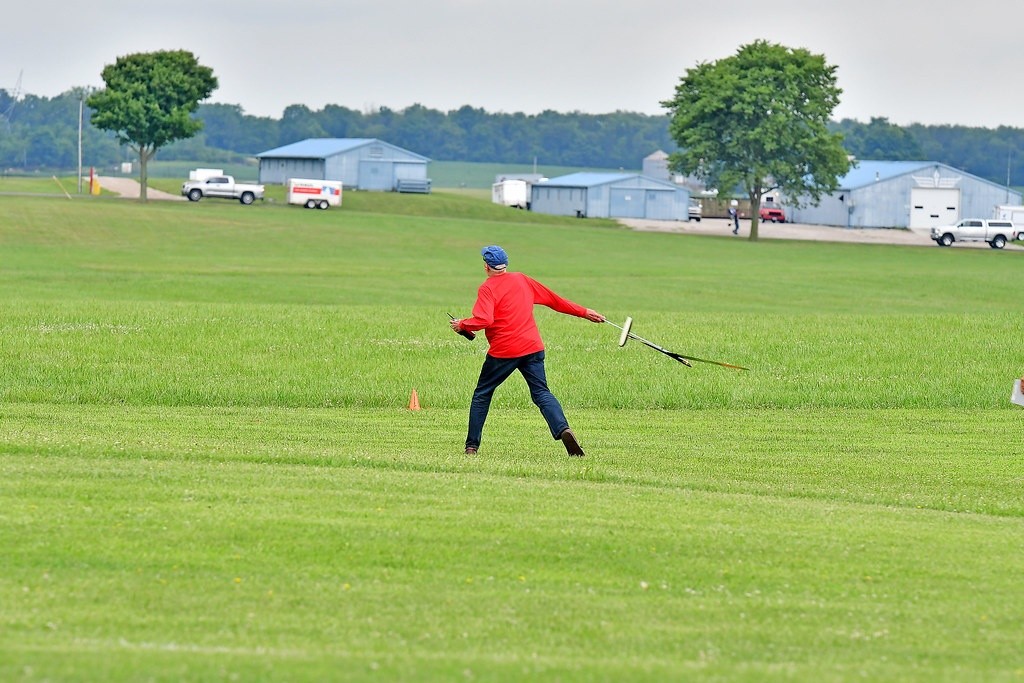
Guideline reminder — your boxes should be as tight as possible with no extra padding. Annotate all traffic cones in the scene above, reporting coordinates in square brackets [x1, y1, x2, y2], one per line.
[407, 390, 421, 410]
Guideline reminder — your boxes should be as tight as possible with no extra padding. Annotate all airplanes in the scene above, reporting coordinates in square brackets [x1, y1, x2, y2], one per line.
[605, 316, 752, 372]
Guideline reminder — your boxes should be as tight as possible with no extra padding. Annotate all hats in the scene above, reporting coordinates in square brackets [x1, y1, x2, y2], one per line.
[481, 246, 508, 270]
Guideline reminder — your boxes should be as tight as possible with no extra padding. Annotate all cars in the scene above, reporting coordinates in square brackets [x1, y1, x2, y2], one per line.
[1015, 224, 1024, 241]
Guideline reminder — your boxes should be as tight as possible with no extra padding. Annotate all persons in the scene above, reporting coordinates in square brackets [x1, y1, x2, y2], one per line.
[728, 206, 739, 234]
[449, 246, 606, 456]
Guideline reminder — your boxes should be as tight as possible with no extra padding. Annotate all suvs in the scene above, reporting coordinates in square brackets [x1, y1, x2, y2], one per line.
[931, 218, 1015, 249]
[757, 203, 785, 223]
[688, 197, 703, 221]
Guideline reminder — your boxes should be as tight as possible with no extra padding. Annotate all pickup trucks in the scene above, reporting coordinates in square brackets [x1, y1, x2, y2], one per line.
[182, 175, 265, 205]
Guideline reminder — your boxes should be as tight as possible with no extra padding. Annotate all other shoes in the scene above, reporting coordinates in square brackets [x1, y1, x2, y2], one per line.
[465, 447, 477, 454]
[561, 429, 585, 457]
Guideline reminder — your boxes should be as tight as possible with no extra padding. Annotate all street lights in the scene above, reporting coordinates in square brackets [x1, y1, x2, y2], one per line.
[76, 97, 84, 192]
